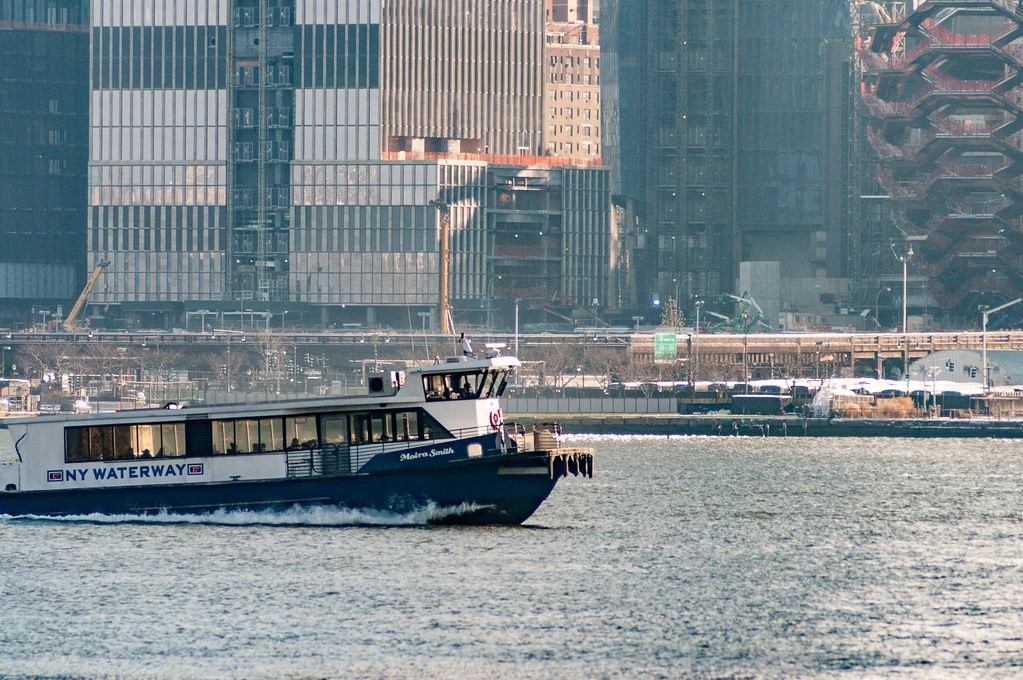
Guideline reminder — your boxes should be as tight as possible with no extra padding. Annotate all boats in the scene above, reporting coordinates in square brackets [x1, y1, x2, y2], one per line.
[0, 327, 596, 532]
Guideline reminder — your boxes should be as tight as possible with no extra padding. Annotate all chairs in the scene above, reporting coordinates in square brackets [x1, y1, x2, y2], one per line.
[443, 387, 458, 399]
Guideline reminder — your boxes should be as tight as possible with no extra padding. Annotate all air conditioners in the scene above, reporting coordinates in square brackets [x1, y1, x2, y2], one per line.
[368, 371, 397, 396]
[396, 371, 405, 391]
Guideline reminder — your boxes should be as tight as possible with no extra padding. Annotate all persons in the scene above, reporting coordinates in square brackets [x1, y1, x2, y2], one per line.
[226, 443, 234, 454]
[156, 447, 162, 458]
[125, 448, 135, 458]
[457, 333, 466, 356]
[140, 449, 152, 458]
[460, 383, 472, 396]
[250, 443, 267, 452]
[289, 438, 318, 449]
[102, 448, 113, 459]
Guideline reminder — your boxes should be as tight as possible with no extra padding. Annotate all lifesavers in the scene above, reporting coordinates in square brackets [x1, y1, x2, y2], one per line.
[489, 411, 500, 429]
[498, 408, 504, 423]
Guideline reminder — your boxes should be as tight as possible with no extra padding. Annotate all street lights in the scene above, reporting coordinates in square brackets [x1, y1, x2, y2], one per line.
[875, 287, 892, 330]
[485, 274, 503, 333]
[631, 315, 644, 333]
[696, 299, 706, 381]
[900, 248, 917, 384]
[677, 276, 693, 309]
